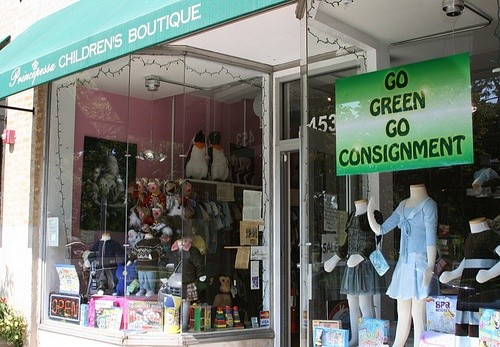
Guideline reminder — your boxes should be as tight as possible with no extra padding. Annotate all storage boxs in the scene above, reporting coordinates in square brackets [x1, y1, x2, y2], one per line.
[83, 296, 164, 335]
[312, 318, 391, 347]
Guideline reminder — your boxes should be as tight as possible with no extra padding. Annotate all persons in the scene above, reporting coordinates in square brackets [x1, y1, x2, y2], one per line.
[159, 239, 206, 330]
[126, 233, 168, 297]
[439, 217, 500, 347]
[324, 200, 383, 347]
[87, 233, 129, 295]
[367, 184, 439, 347]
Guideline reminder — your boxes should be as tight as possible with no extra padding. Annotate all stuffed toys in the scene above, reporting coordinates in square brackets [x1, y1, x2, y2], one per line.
[127, 177, 193, 244]
[207, 131, 231, 181]
[185, 130, 209, 180]
[206, 275, 241, 309]
